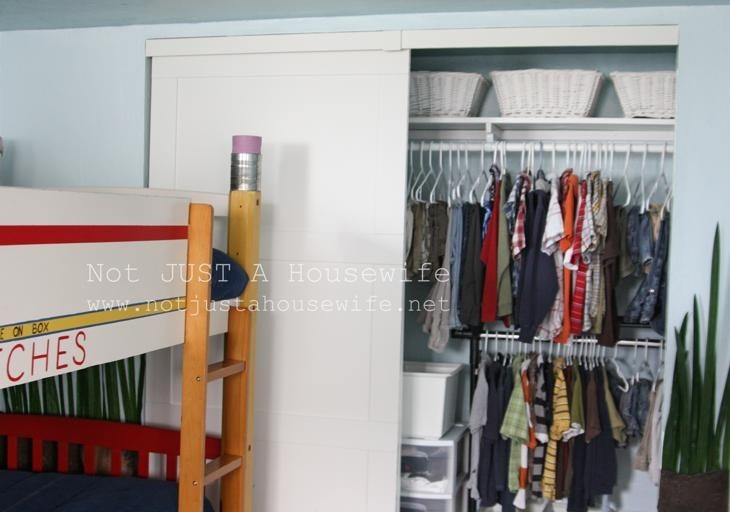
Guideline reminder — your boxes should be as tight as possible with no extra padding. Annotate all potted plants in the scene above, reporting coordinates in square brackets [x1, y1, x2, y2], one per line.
[657, 221, 729, 512]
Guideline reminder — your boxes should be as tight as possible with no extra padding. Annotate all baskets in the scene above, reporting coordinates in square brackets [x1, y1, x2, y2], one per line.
[489, 69, 605, 117]
[410, 70, 489, 117]
[609, 71, 676, 118]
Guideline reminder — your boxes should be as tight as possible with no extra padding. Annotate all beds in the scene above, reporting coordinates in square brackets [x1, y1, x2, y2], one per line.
[0, 136, 260, 511]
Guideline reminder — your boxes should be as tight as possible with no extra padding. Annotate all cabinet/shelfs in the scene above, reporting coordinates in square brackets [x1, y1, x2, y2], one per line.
[142, 26, 678, 512]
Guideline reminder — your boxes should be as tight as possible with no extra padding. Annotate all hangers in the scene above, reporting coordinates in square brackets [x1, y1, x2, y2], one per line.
[407, 141, 675, 222]
[473, 330, 667, 393]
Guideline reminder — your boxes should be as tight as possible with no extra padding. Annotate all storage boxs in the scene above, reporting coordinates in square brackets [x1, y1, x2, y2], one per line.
[410, 69, 676, 117]
[400, 361, 470, 512]
